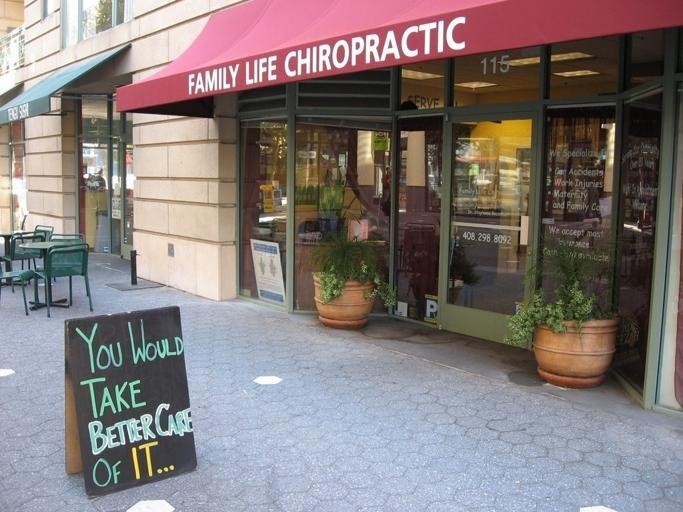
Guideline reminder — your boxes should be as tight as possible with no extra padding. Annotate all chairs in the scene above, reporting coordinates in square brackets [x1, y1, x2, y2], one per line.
[0, 224, 94, 319]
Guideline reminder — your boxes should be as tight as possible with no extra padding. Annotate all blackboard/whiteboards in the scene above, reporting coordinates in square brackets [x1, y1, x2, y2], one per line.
[65, 305, 197, 499]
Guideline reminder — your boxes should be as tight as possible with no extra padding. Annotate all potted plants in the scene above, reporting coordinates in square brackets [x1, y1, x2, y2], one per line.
[306, 235, 398, 330]
[500, 229, 621, 388]
[405, 235, 483, 314]
[314, 185, 346, 239]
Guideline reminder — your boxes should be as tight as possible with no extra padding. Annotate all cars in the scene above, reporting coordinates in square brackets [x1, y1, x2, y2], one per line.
[472, 176, 507, 191]
